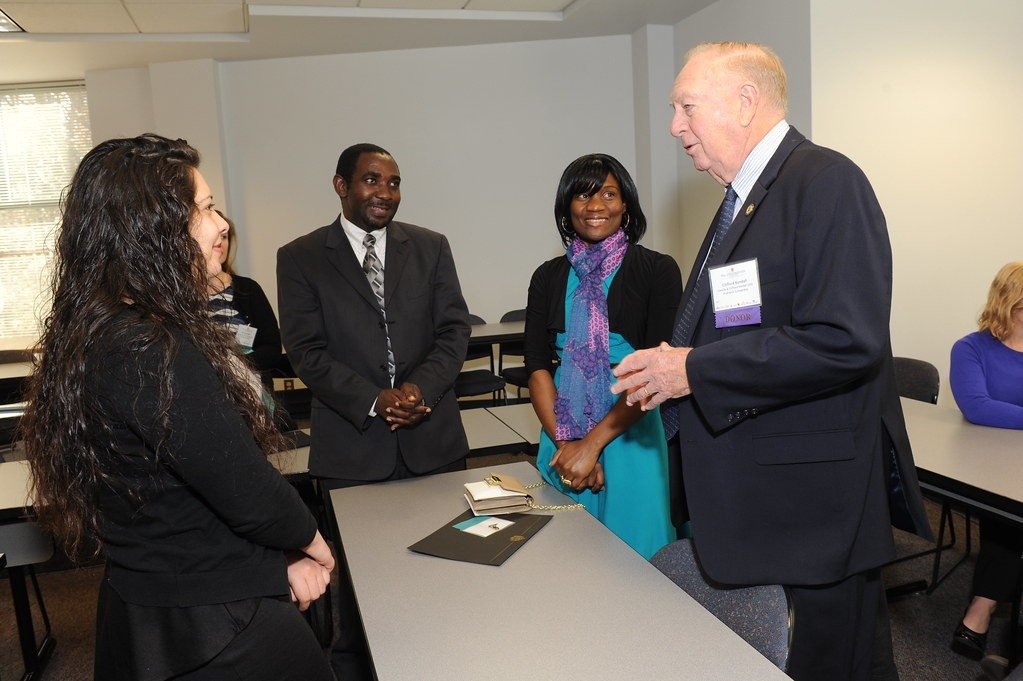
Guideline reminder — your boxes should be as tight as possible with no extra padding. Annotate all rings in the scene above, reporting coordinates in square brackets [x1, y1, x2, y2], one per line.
[643, 385, 650, 397]
[561, 479, 571, 485]
[557, 473, 563, 479]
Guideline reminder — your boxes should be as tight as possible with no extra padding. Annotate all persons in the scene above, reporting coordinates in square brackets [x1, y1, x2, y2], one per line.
[208, 210, 280, 397]
[275, 144, 472, 661]
[949, 262, 1023, 679]
[524, 154, 683, 564]
[611, 39, 932, 681]
[28, 134, 334, 681]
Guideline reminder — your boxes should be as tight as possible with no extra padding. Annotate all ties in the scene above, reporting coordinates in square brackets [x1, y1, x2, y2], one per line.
[659, 183, 737, 442]
[363, 233, 396, 390]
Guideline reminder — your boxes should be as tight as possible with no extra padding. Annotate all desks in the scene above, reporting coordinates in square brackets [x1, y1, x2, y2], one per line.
[885, 396, 1023, 598]
[0, 362, 542, 681]
[470, 321, 526, 344]
[330, 460, 796, 681]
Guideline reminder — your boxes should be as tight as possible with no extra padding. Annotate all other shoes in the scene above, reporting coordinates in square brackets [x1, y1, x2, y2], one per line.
[951, 603, 992, 661]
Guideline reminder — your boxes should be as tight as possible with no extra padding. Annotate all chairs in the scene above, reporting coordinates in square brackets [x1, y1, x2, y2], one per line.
[882, 355, 956, 566]
[455, 306, 529, 410]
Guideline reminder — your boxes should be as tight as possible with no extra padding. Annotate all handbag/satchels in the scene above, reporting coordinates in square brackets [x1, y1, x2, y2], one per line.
[464, 472, 584, 516]
